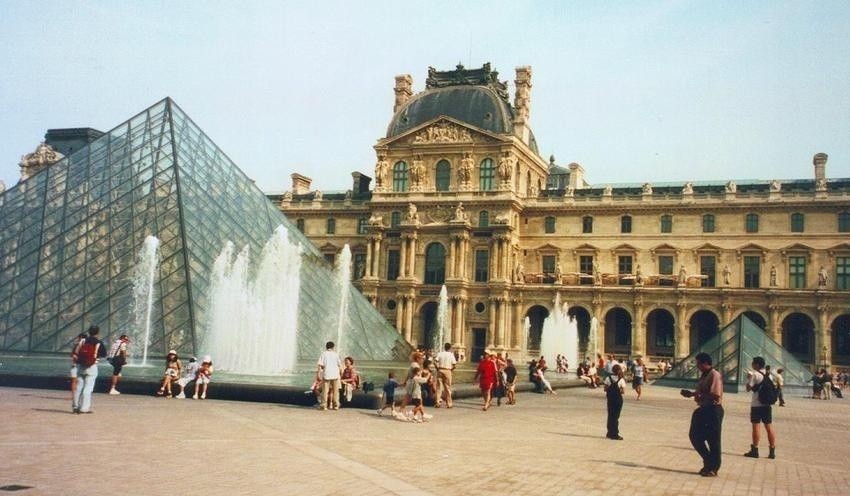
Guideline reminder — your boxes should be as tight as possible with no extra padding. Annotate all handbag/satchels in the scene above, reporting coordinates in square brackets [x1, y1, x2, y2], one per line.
[114, 355, 126, 365]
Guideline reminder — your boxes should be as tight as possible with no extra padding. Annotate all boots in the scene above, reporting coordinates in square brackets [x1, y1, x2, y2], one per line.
[768, 446, 775, 459]
[745, 445, 758, 458]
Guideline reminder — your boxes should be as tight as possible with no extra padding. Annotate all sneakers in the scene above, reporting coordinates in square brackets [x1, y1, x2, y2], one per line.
[377, 408, 434, 422]
[157, 388, 206, 399]
[110, 388, 120, 395]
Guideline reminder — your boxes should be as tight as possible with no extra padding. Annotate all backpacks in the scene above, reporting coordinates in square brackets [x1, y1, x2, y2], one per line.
[79, 343, 96, 365]
[757, 370, 776, 404]
[607, 375, 621, 402]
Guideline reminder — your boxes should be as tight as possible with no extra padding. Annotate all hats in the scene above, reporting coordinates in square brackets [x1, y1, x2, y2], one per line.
[169, 350, 176, 355]
[204, 356, 211, 363]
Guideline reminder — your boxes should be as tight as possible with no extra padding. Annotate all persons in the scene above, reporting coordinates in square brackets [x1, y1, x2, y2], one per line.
[775, 368, 785, 408]
[631, 358, 648, 400]
[72, 325, 106, 414]
[804, 369, 849, 401]
[474, 350, 517, 411]
[156, 349, 181, 398]
[603, 363, 626, 441]
[658, 359, 672, 374]
[174, 357, 200, 399]
[313, 342, 357, 411]
[530, 356, 555, 394]
[744, 356, 776, 459]
[378, 343, 457, 423]
[577, 353, 627, 387]
[557, 355, 568, 373]
[191, 356, 212, 400]
[680, 352, 726, 477]
[106, 335, 130, 397]
[70, 332, 88, 397]
